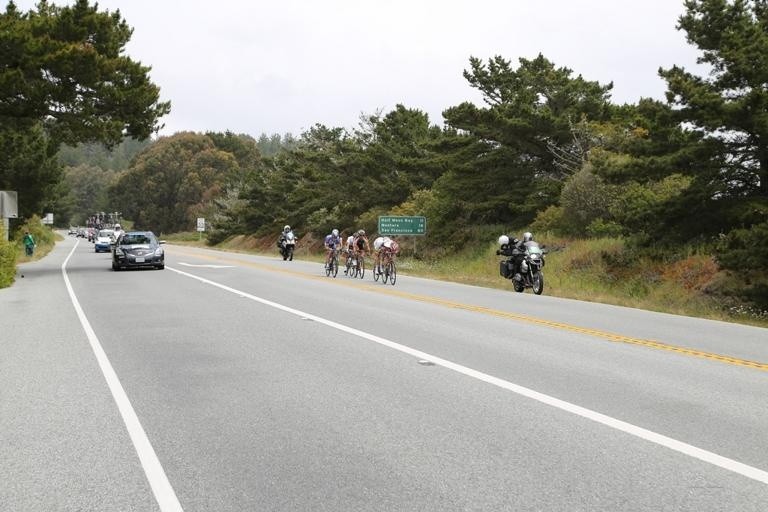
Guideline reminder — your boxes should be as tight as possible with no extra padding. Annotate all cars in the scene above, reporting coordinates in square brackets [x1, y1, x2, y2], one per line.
[68, 226, 113, 252]
[111, 231, 165, 270]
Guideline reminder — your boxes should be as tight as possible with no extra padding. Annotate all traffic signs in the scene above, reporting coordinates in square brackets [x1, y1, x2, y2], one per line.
[378, 216, 426, 237]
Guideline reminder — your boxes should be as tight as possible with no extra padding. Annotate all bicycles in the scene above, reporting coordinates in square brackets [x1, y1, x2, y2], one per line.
[325, 248, 342, 277]
[344, 248, 369, 278]
[374, 249, 396, 285]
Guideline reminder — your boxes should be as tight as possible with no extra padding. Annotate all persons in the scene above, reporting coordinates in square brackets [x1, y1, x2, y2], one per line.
[510, 231, 547, 282]
[280, 224, 298, 256]
[111, 223, 125, 241]
[22, 231, 35, 257]
[323, 226, 400, 276]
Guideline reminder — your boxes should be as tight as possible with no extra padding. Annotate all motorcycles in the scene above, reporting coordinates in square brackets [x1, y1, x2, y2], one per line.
[500, 241, 549, 295]
[277, 231, 299, 261]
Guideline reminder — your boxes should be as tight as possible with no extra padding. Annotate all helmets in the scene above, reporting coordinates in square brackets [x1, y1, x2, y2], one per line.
[332, 229, 339, 238]
[284, 225, 290, 233]
[524, 232, 532, 242]
[358, 230, 365, 239]
[383, 237, 390, 241]
[390, 243, 398, 252]
[499, 235, 509, 250]
[353, 233, 359, 238]
